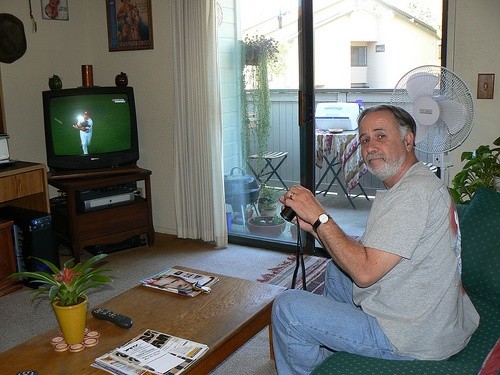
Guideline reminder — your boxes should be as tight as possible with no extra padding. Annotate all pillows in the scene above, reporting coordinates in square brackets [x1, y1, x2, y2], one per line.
[458, 188, 499, 305]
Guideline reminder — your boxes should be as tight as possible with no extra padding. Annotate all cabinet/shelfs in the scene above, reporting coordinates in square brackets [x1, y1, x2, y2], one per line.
[48, 164, 159, 262]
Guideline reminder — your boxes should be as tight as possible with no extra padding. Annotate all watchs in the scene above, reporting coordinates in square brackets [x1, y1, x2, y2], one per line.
[313, 213, 332, 232]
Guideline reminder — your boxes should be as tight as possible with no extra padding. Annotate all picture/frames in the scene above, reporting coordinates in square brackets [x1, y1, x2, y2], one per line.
[105, 0, 154, 52]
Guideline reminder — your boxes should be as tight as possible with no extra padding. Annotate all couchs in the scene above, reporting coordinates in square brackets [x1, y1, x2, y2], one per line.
[312, 190, 500, 375]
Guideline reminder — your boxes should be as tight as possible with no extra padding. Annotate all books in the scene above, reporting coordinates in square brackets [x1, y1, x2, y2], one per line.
[139, 267, 219, 297]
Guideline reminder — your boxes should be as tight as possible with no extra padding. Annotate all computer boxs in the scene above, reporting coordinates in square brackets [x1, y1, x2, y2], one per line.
[0, 207, 60, 289]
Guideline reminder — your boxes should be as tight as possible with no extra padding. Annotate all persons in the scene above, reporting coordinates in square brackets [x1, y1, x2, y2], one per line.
[271, 104, 480, 375]
[151, 275, 195, 294]
[73, 112, 93, 154]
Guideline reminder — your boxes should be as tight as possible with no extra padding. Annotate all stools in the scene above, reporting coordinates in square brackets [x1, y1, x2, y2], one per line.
[0, 219, 25, 297]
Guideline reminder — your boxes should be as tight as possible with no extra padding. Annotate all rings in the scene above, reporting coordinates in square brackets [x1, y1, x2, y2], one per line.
[290, 195, 293, 199]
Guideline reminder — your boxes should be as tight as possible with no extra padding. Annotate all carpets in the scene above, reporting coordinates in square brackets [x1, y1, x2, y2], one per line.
[257, 254, 328, 296]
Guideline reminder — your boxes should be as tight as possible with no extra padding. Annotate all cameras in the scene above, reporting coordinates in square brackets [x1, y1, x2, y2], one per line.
[280, 206, 296, 221]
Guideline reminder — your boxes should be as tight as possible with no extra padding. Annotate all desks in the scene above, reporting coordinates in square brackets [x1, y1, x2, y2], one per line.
[315, 134, 370, 210]
[0, 161, 52, 213]
[247, 151, 291, 191]
[0, 265, 289, 375]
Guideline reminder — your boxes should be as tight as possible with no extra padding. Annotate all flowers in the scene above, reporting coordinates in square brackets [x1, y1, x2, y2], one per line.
[8, 254, 116, 308]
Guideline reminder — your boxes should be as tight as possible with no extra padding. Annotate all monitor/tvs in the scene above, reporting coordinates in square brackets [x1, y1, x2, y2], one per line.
[42, 86, 139, 171]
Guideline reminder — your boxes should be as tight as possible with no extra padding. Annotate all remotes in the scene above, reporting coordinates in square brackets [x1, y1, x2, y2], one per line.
[91, 307, 133, 329]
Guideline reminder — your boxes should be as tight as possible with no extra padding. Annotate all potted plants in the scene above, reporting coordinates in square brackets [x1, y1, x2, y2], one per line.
[247, 215, 286, 239]
[255, 182, 282, 216]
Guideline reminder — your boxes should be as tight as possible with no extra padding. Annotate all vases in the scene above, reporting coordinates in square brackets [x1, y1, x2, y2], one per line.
[52, 296, 88, 345]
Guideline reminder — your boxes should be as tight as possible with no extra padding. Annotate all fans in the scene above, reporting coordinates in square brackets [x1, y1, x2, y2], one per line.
[391, 65, 474, 186]
